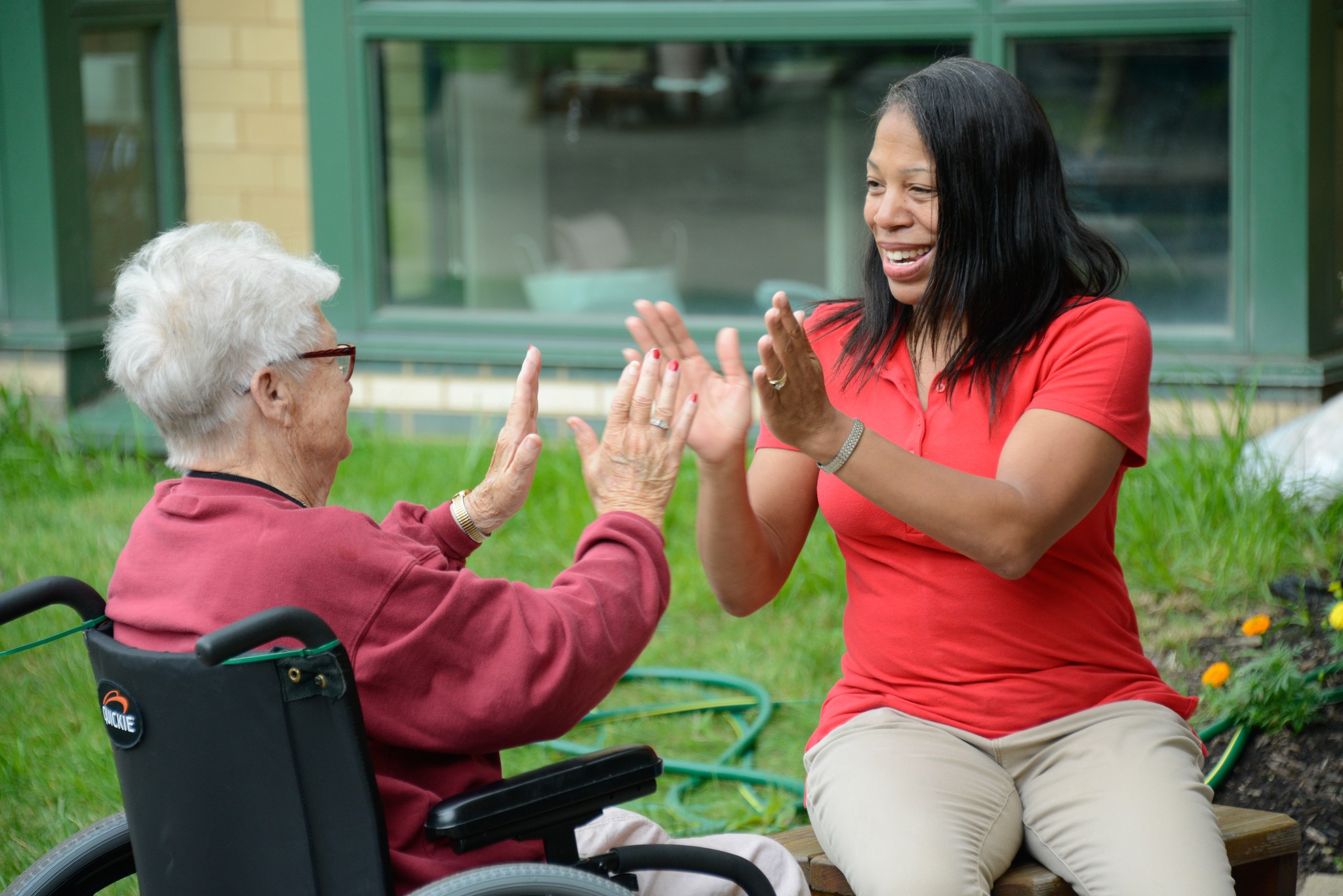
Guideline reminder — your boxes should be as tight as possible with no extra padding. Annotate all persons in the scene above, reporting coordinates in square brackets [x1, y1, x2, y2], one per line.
[106, 220, 811, 896]
[624, 52, 1240, 896]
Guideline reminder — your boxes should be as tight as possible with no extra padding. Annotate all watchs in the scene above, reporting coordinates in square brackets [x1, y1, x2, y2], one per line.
[450, 487, 492, 544]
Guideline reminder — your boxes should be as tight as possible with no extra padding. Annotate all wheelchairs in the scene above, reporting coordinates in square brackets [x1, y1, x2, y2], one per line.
[1, 572, 775, 896]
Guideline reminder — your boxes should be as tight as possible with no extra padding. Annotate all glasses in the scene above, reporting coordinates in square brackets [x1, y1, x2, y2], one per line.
[234, 343, 356, 396]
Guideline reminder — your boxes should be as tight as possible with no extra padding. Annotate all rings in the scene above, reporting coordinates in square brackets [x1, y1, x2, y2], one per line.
[649, 417, 671, 431]
[768, 372, 788, 390]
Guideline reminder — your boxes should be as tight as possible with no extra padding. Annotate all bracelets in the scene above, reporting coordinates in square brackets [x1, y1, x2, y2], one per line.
[819, 416, 864, 477]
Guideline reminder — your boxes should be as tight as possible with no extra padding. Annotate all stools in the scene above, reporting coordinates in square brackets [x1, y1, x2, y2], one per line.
[763, 805, 1301, 896]
